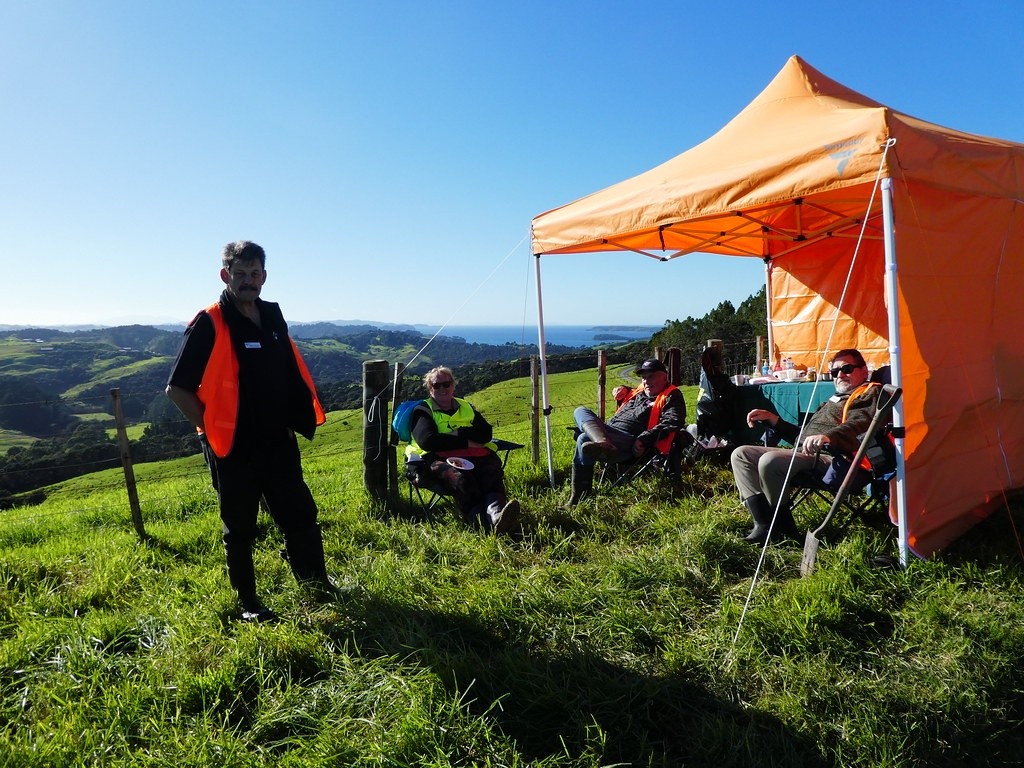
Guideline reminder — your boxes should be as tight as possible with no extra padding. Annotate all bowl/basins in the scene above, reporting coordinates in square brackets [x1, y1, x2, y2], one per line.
[822, 373, 833, 381]
[445, 456, 475, 472]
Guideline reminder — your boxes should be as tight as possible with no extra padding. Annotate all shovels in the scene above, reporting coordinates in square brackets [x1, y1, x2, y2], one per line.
[800, 383, 904, 578]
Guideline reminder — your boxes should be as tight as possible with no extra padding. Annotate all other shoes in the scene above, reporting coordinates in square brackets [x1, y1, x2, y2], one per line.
[316, 584, 352, 603]
[238, 598, 273, 621]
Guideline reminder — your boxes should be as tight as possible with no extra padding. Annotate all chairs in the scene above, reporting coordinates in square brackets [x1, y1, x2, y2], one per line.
[695, 352, 779, 467]
[790, 366, 892, 539]
[566, 386, 695, 502]
[406, 437, 526, 529]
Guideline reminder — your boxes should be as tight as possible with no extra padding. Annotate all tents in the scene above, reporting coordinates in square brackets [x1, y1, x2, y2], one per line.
[528, 57, 1024, 571]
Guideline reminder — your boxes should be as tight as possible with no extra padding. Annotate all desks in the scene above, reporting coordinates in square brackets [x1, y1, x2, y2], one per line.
[757, 379, 836, 447]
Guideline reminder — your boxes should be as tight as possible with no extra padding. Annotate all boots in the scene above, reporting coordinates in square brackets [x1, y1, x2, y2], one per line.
[486, 498, 520, 537]
[582, 421, 619, 459]
[744, 493, 803, 551]
[563, 463, 594, 509]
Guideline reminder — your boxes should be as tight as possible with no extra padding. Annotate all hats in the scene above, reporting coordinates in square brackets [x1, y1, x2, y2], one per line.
[635, 359, 668, 378]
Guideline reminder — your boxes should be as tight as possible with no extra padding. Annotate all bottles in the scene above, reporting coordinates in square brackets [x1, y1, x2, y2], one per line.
[807, 367, 817, 383]
[768, 356, 795, 375]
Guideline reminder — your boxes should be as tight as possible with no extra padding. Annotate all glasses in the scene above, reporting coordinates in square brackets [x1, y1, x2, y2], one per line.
[431, 381, 453, 389]
[831, 364, 860, 378]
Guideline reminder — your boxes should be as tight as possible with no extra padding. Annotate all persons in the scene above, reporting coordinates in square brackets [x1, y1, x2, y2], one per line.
[407, 367, 521, 536]
[697, 348, 762, 450]
[731, 349, 896, 549]
[165, 241, 346, 622]
[565, 358, 686, 510]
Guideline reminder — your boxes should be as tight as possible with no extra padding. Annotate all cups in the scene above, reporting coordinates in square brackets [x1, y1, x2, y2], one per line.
[734, 375, 744, 386]
